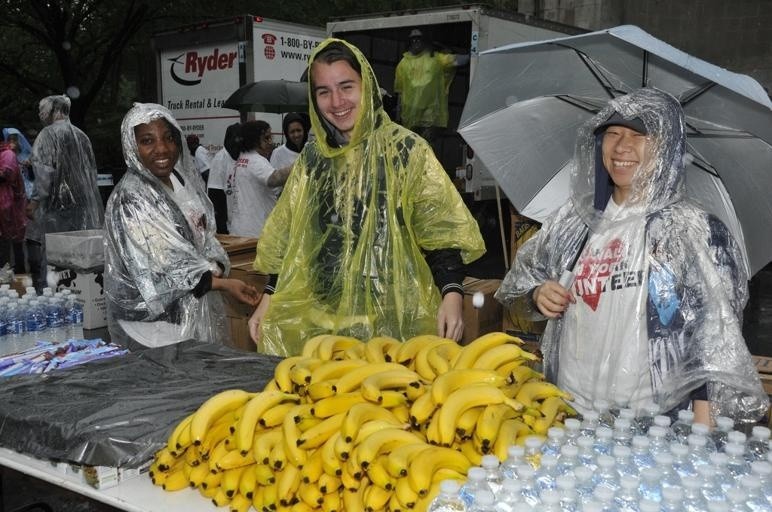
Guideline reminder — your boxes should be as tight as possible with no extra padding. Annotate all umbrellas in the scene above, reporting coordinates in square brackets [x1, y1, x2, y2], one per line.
[85, 126, 117, 167]
[219, 77, 310, 148]
[454, 24, 772, 320]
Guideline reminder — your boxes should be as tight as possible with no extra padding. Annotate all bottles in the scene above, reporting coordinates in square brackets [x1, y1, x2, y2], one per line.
[426, 398, 772, 511]
[0, 284, 84, 361]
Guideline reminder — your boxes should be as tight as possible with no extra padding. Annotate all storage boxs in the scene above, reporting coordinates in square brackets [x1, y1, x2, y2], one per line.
[750, 355, 771, 429]
[462, 203, 543, 345]
[45, 231, 107, 330]
[216, 234, 272, 352]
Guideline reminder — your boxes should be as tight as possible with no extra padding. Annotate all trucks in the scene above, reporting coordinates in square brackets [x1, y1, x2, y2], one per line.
[149, 15, 324, 153]
[324, 6, 611, 204]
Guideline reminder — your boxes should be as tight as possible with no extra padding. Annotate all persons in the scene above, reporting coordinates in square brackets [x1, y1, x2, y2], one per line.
[186, 110, 308, 240]
[102, 103, 262, 354]
[494, 87, 748, 455]
[4, 129, 38, 272]
[81, 112, 117, 176]
[393, 29, 469, 149]
[1, 128, 26, 276]
[20, 94, 103, 289]
[246, 39, 467, 359]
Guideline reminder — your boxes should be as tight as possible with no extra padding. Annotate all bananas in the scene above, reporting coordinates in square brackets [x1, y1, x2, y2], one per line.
[150, 332, 581, 512]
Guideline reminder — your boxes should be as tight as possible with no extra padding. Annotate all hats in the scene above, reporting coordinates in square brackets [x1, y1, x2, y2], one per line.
[591, 107, 650, 136]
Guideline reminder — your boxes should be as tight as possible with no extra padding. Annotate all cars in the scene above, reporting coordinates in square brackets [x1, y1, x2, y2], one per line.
[96, 169, 114, 186]
